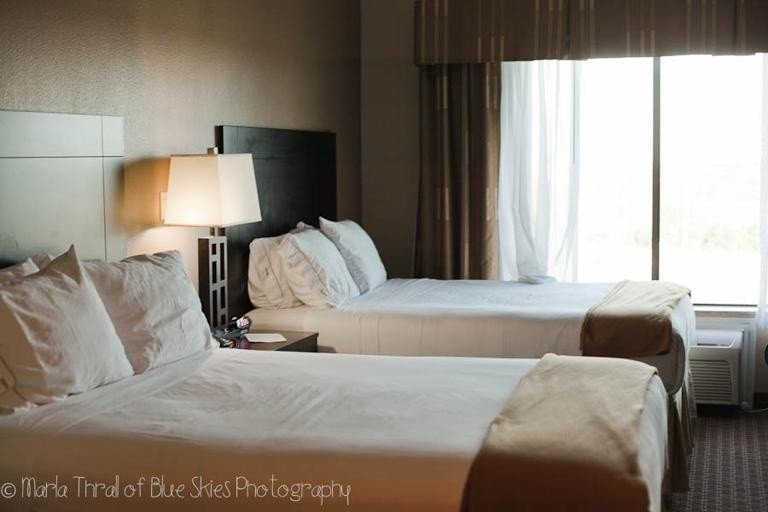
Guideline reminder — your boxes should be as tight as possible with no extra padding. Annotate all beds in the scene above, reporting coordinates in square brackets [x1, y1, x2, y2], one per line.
[213, 118, 701, 495]
[2, 110, 692, 510]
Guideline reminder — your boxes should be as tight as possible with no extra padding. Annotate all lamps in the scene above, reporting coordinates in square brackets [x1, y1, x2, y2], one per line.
[162, 144, 266, 331]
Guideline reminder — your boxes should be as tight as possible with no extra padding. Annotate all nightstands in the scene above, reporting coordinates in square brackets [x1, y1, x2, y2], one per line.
[215, 323, 322, 352]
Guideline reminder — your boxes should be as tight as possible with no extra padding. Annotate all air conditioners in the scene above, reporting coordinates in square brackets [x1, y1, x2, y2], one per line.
[687, 327, 746, 408]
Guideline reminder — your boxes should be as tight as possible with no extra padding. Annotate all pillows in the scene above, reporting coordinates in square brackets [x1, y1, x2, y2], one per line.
[280, 220, 359, 312]
[318, 212, 386, 296]
[1, 257, 45, 414]
[0, 243, 135, 406]
[42, 248, 221, 372]
[246, 230, 298, 312]
